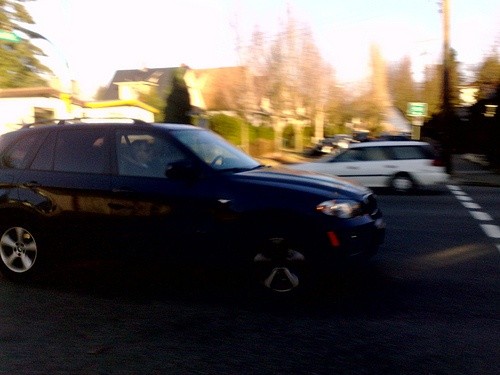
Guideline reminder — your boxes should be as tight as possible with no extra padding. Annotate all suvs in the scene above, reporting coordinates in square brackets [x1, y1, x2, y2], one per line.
[0, 117, 383, 294]
[282, 141, 450, 192]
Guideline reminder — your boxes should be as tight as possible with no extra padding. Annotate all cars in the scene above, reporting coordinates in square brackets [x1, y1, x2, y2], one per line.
[312, 129, 425, 152]
